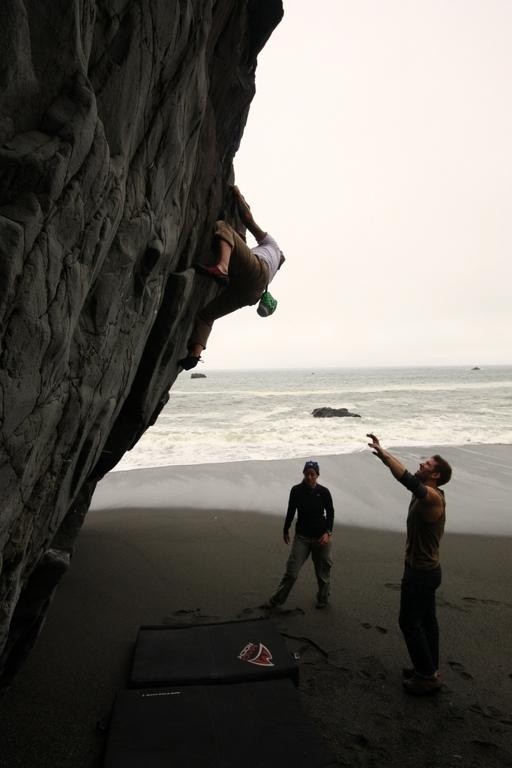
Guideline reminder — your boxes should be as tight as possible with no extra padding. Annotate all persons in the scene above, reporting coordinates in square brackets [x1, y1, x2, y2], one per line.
[177, 184, 285, 369]
[366, 434, 454, 689]
[257, 461, 335, 610]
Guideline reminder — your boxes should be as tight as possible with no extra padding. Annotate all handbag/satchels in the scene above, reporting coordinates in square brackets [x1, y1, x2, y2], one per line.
[256, 291, 277, 317]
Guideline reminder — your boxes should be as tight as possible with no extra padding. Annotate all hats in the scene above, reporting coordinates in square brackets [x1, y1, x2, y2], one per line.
[302, 461, 319, 475]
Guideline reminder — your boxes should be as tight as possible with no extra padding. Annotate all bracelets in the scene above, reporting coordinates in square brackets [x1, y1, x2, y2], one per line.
[326, 531, 331, 536]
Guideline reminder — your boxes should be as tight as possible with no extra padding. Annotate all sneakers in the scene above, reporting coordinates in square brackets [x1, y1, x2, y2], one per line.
[194, 262, 230, 285]
[400, 666, 441, 691]
[176, 354, 199, 370]
